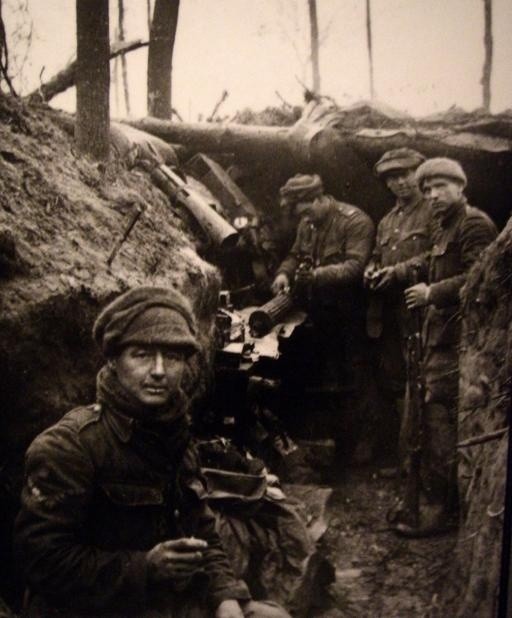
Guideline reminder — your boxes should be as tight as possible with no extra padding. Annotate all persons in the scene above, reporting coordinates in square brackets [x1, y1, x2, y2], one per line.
[362, 145, 442, 478]
[396, 154, 500, 536]
[15, 286, 290, 618]
[271, 171, 380, 479]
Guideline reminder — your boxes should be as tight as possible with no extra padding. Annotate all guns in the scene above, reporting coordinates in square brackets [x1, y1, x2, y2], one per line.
[395, 263, 429, 527]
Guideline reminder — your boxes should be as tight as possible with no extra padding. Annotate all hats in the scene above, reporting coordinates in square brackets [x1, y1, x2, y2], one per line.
[416, 157, 467, 186]
[93, 286, 204, 355]
[374, 149, 420, 177]
[280, 173, 323, 205]
